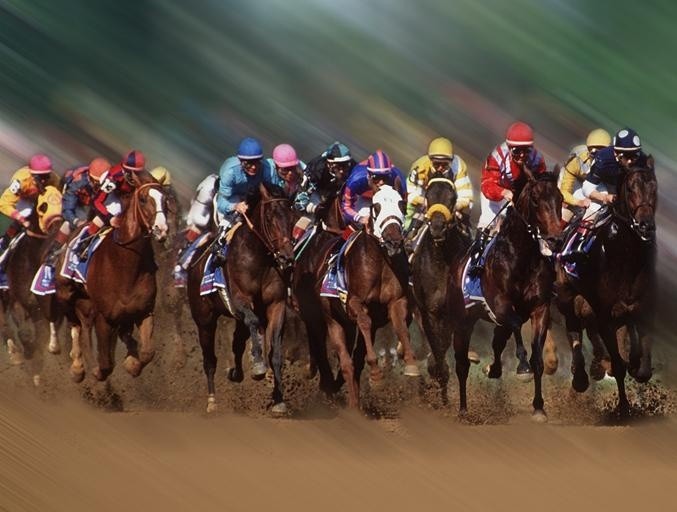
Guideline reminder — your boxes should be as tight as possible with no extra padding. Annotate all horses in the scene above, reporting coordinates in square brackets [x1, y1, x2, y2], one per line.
[288, 177, 420, 407]
[412, 165, 476, 413]
[0, 176, 66, 387]
[304, 198, 377, 392]
[156, 193, 181, 354]
[186, 183, 296, 413]
[261, 266, 303, 360]
[448, 161, 565, 421]
[555, 154, 657, 415]
[55, 168, 171, 382]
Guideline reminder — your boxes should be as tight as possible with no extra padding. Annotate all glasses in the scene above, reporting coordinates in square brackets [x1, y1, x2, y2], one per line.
[328, 163, 351, 171]
[242, 160, 261, 168]
[617, 153, 640, 160]
[371, 174, 391, 180]
[279, 168, 297, 174]
[589, 149, 600, 156]
[432, 159, 450, 167]
[510, 148, 531, 154]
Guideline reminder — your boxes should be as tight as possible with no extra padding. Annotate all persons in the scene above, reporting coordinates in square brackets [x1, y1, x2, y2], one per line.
[399, 136, 475, 263]
[214, 136, 272, 260]
[149, 167, 174, 188]
[561, 126, 647, 281]
[465, 121, 548, 300]
[267, 142, 306, 188]
[45, 157, 114, 269]
[554, 129, 612, 233]
[74, 149, 145, 260]
[0, 154, 60, 293]
[291, 140, 361, 245]
[319, 149, 407, 299]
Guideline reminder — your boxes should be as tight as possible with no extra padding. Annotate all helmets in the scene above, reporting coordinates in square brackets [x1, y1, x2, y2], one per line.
[613, 128, 641, 152]
[89, 159, 112, 181]
[367, 151, 392, 175]
[273, 144, 299, 168]
[29, 155, 51, 175]
[237, 137, 264, 160]
[587, 128, 611, 148]
[428, 137, 453, 160]
[507, 123, 534, 147]
[122, 150, 145, 171]
[150, 166, 172, 192]
[326, 142, 352, 162]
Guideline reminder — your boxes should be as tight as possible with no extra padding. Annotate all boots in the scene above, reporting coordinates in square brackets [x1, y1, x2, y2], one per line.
[467, 228, 490, 274]
[76, 231, 91, 261]
[568, 221, 591, 254]
[328, 237, 346, 274]
[0, 232, 11, 257]
[45, 241, 60, 268]
[213, 226, 231, 265]
[177, 241, 193, 272]
[403, 218, 423, 253]
